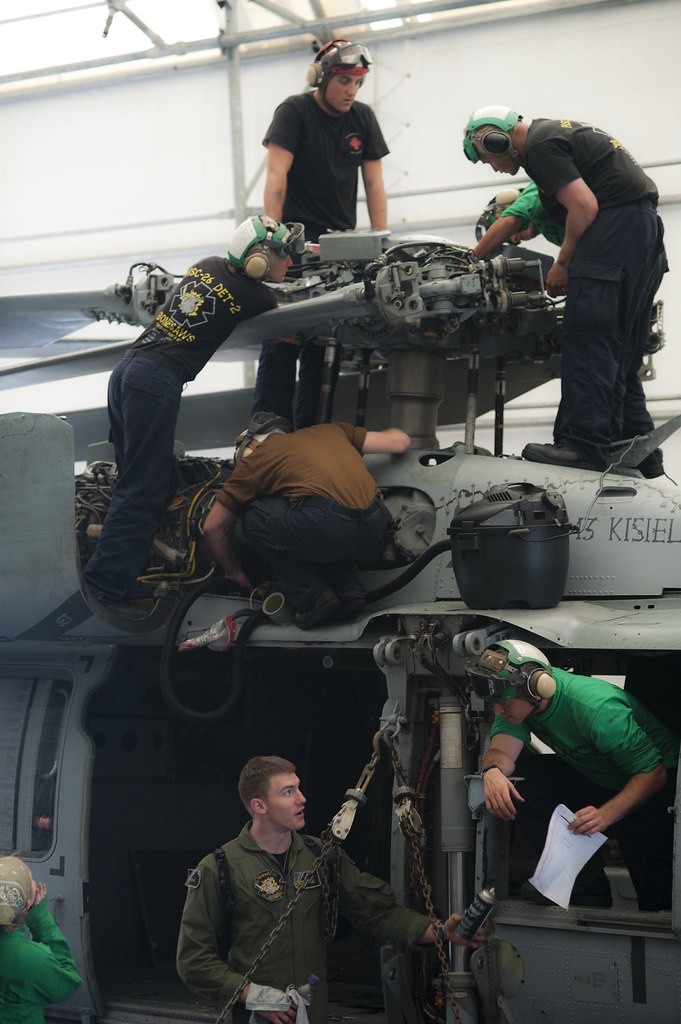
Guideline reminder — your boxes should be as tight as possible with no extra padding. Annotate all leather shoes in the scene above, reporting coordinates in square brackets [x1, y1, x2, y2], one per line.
[637, 450, 665, 479]
[522, 441, 609, 472]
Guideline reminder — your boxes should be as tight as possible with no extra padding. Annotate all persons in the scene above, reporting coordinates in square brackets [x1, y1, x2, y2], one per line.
[0, 856, 82, 1024]
[463, 104, 656, 472]
[464, 640, 681, 913]
[82, 215, 307, 602]
[204, 411, 410, 630]
[470, 179, 671, 479]
[248, 40, 390, 431]
[176, 756, 486, 1024]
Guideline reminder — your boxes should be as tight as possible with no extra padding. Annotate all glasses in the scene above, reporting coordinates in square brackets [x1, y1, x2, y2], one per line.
[466, 672, 512, 699]
[284, 222, 306, 256]
[237, 411, 293, 460]
[323, 43, 373, 70]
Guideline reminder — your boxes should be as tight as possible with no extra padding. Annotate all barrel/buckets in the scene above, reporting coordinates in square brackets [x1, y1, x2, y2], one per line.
[446, 522, 580, 611]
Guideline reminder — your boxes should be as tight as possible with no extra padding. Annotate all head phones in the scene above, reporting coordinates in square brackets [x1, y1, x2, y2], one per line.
[479, 650, 559, 701]
[242, 213, 280, 283]
[234, 435, 252, 465]
[307, 39, 346, 87]
[464, 131, 513, 157]
[483, 189, 521, 212]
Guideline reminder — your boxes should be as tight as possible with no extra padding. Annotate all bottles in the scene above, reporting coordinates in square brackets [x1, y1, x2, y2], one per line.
[287, 973, 319, 1009]
[455, 886, 496, 944]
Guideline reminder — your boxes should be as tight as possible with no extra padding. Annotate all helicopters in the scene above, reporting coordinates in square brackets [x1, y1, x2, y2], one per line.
[0, 232, 680, 1024]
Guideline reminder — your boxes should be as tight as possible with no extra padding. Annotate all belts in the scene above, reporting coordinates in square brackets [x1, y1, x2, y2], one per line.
[627, 198, 658, 214]
[122, 350, 187, 384]
[302, 492, 382, 517]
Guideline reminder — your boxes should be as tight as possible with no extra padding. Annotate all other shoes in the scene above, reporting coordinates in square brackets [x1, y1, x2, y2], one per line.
[89, 580, 154, 601]
[294, 579, 366, 629]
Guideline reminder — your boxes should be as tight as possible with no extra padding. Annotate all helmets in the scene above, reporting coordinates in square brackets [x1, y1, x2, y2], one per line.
[463, 106, 524, 164]
[233, 428, 286, 460]
[475, 188, 524, 241]
[227, 215, 291, 283]
[307, 37, 373, 99]
[463, 639, 557, 708]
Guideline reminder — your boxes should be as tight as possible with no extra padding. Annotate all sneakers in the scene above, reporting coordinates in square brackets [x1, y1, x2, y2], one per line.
[519, 873, 613, 907]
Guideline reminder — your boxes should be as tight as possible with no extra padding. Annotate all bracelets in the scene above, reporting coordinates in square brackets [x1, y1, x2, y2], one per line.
[481, 763, 504, 776]
[439, 919, 446, 943]
